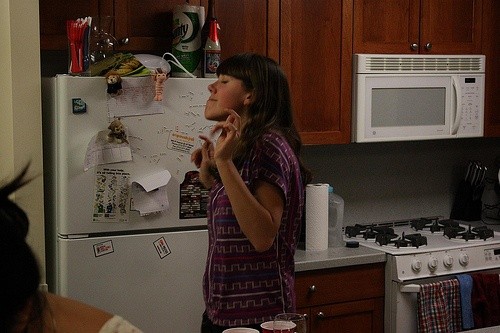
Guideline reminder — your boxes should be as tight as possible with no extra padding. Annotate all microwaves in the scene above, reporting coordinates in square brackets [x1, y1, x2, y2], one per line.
[352, 52, 486, 144]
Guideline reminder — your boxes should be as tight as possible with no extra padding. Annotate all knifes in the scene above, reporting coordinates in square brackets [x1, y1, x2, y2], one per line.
[463, 158, 488, 200]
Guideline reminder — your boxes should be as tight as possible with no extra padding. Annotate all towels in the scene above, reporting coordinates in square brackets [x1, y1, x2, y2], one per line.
[416, 278, 462, 333]
[455, 273, 500, 329]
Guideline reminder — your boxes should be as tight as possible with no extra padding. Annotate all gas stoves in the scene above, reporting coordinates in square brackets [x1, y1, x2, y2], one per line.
[342, 216, 500, 283]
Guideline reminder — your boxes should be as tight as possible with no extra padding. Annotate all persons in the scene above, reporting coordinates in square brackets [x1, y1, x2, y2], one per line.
[191, 52, 313, 333]
[0, 158, 58, 333]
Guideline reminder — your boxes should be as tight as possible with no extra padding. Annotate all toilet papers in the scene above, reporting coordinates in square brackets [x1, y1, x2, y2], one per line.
[305, 183, 329, 251]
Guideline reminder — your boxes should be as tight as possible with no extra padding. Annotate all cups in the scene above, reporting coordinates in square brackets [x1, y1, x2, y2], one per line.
[66, 15, 114, 77]
[222, 327, 260, 333]
[273, 313, 306, 333]
[261, 321, 297, 333]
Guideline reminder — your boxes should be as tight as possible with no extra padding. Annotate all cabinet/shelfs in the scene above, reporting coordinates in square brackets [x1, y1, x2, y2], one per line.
[352, 0, 482, 55]
[293, 264, 386, 333]
[201, 0, 353, 146]
[39, 0, 200, 53]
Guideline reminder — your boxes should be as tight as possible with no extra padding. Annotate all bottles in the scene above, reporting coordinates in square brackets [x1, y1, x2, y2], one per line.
[203, 17, 222, 78]
[201, 0, 222, 76]
[481, 176, 500, 225]
[328, 185, 345, 248]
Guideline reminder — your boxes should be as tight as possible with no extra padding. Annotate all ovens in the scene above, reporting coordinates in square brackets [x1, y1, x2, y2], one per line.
[383, 268, 500, 333]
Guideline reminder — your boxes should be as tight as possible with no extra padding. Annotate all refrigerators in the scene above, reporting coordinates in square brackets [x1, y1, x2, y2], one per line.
[42, 73, 219, 333]
